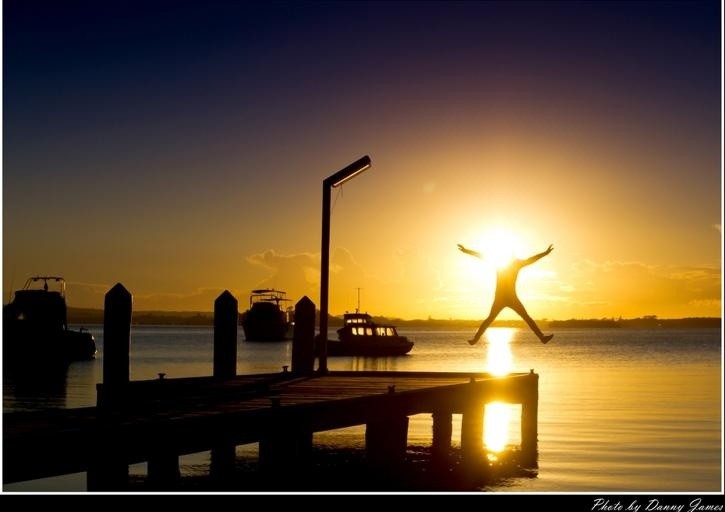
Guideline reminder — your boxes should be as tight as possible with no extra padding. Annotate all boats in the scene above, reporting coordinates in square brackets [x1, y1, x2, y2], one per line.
[314, 309, 415, 357]
[2, 276, 96, 362]
[241, 289, 296, 341]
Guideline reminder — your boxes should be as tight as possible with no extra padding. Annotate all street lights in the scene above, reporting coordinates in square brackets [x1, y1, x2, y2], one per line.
[319, 154, 372, 375]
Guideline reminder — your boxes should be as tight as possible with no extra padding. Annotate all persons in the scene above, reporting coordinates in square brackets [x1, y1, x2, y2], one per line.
[456, 244, 557, 349]
